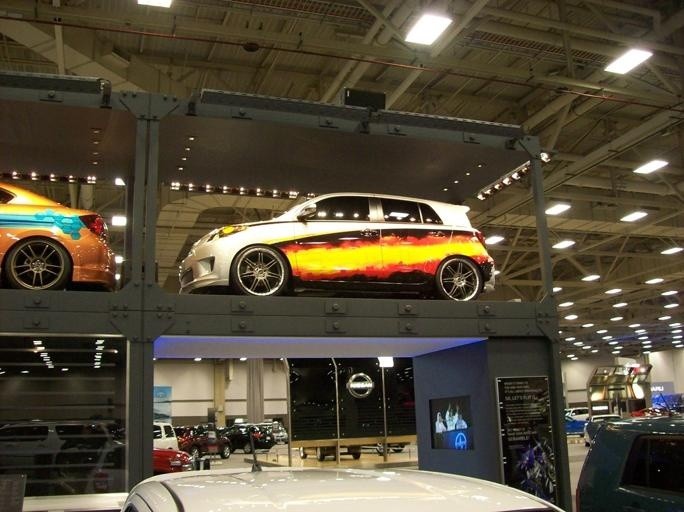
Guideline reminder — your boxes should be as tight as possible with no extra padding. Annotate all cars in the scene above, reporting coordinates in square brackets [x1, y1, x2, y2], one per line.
[0, 182, 119, 290]
[175, 193, 496, 303]
[563, 393, 683, 511]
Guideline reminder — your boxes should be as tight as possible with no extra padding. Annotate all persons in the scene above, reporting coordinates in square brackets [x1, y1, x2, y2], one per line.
[445, 402, 460, 431]
[454, 410, 467, 430]
[432, 411, 446, 433]
